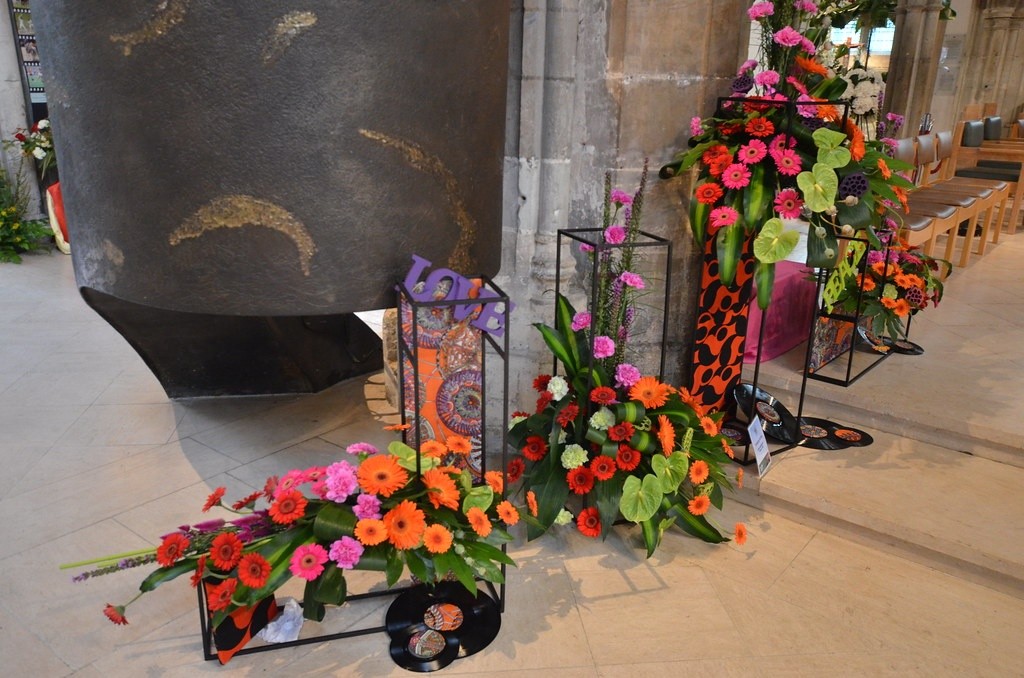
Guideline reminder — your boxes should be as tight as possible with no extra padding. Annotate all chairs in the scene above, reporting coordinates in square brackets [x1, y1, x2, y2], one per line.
[916, 134, 997, 256]
[978, 103, 1024, 171]
[947, 105, 1024, 234]
[901, 202, 956, 263]
[897, 209, 936, 256]
[935, 130, 1010, 244]
[892, 136, 978, 266]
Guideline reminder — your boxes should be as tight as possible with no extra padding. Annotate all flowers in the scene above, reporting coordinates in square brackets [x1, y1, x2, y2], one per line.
[2, 115, 57, 180]
[507, 156, 749, 559]
[0, 158, 55, 265]
[658, 0, 958, 308]
[58, 435, 539, 625]
[804, 234, 951, 344]
